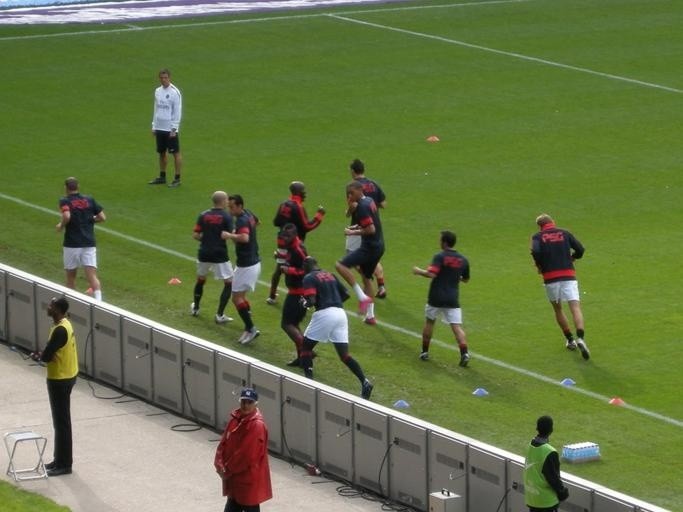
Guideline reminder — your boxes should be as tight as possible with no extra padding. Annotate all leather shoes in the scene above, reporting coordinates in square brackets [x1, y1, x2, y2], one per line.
[45, 462, 71, 475]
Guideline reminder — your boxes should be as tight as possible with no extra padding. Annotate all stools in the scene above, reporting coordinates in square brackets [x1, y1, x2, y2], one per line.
[3, 429, 50, 484]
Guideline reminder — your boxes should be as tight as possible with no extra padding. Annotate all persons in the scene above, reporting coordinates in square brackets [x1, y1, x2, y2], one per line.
[146, 68, 182, 187]
[296, 255, 373, 399]
[530, 213, 591, 360]
[344, 158, 385, 298]
[523, 414, 568, 511]
[219, 194, 261, 345]
[334, 181, 385, 324]
[55, 178, 107, 301]
[213, 387, 273, 511]
[264, 181, 325, 304]
[31, 296, 78, 476]
[188, 191, 234, 323]
[410, 231, 471, 368]
[272, 222, 315, 367]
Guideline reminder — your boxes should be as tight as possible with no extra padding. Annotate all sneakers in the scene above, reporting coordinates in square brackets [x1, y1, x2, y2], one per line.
[149, 177, 166, 183]
[360, 296, 372, 311]
[288, 351, 316, 378]
[215, 313, 233, 322]
[566, 340, 577, 350]
[361, 377, 373, 399]
[460, 353, 470, 366]
[168, 180, 181, 187]
[239, 327, 259, 345]
[577, 338, 590, 360]
[375, 288, 386, 297]
[421, 352, 428, 360]
[364, 317, 375, 324]
[192, 302, 199, 316]
[266, 294, 279, 303]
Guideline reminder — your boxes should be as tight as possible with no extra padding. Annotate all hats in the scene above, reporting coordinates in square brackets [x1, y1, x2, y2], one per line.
[240, 389, 257, 401]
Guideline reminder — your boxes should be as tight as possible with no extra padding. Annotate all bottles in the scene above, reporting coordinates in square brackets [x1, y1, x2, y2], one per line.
[562, 441, 600, 464]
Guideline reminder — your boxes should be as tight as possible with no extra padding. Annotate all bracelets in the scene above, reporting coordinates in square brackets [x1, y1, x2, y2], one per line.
[302, 301, 306, 308]
